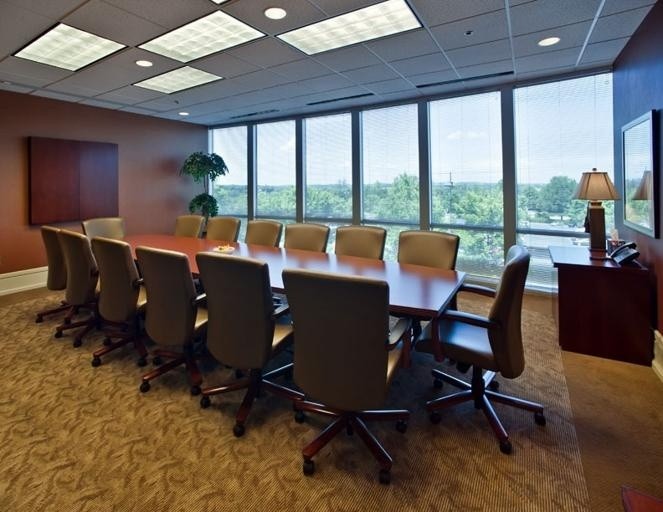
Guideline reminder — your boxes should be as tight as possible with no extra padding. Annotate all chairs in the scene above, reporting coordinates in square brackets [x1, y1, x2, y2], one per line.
[280, 266, 411, 477]
[194, 249, 306, 438]
[413, 245, 545, 456]
[397, 229, 460, 271]
[244, 218, 283, 248]
[54, 227, 100, 347]
[81, 216, 126, 239]
[284, 223, 330, 253]
[206, 216, 241, 241]
[136, 240, 209, 396]
[89, 235, 151, 369]
[334, 225, 387, 260]
[174, 215, 206, 238]
[34, 224, 72, 323]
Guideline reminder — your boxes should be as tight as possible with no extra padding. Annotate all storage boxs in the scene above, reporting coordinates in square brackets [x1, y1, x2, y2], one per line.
[606, 239, 626, 256]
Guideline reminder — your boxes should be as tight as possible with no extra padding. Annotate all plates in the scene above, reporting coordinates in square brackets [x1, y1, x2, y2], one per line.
[211, 246, 235, 254]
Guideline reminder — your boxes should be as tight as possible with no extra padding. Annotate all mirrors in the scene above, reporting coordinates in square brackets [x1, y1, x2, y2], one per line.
[620, 109, 659, 239]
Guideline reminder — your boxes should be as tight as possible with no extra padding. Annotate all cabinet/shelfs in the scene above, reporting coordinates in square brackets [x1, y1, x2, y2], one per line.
[27, 135, 119, 226]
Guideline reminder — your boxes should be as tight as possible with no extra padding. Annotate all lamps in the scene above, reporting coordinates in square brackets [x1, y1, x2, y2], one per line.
[570, 168, 624, 252]
[631, 169, 653, 201]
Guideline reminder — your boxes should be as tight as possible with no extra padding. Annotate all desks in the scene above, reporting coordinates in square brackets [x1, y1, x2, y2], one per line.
[108, 233, 465, 327]
[548, 245, 655, 369]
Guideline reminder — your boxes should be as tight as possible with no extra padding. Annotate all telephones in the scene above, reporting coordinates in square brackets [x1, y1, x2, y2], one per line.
[611, 241, 640, 265]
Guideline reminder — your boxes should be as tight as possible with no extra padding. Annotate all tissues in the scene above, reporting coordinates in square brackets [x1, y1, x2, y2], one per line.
[607, 228, 626, 255]
